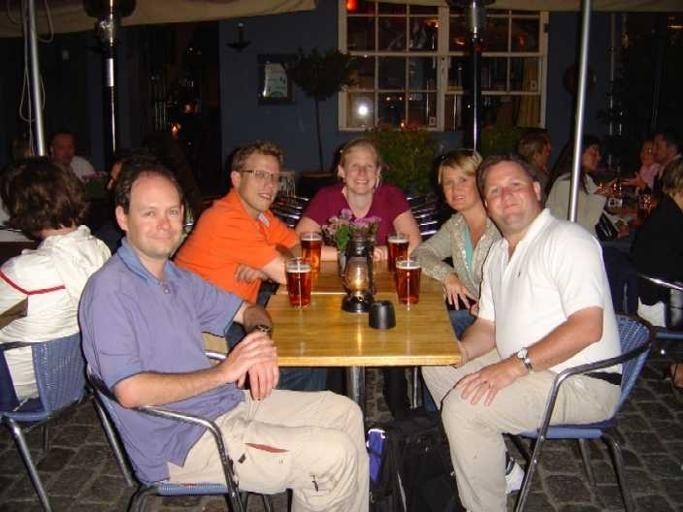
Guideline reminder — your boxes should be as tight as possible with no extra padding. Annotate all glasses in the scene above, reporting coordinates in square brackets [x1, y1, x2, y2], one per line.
[240, 167, 280, 183]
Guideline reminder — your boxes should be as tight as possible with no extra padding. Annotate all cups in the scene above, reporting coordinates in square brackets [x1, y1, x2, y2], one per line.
[301, 229, 323, 273]
[394, 253, 423, 305]
[284, 256, 312, 307]
[386, 230, 411, 274]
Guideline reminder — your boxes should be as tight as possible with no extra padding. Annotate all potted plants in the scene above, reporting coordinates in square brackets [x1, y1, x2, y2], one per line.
[291, 42, 361, 196]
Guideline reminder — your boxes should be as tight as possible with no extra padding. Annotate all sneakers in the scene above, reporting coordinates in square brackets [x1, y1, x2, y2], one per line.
[504, 458, 527, 495]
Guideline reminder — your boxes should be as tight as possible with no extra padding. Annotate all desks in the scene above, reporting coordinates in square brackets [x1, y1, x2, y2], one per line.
[260, 293, 462, 447]
[275, 259, 445, 295]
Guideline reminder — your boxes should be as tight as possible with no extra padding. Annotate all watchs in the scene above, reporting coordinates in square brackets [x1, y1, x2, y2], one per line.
[516, 347, 534, 375]
[248, 323, 272, 338]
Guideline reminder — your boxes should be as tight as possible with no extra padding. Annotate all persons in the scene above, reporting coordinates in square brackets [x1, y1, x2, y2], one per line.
[173, 136, 335, 391]
[407, 147, 504, 413]
[75, 162, 369, 511]
[2, 127, 124, 413]
[292, 137, 423, 257]
[419, 151, 622, 512]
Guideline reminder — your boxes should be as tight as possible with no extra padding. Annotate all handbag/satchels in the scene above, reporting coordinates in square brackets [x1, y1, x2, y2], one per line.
[364, 401, 454, 512]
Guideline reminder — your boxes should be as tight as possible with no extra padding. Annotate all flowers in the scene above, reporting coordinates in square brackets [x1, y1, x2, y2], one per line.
[320, 207, 382, 253]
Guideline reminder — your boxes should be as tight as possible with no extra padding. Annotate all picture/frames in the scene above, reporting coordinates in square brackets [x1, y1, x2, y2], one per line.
[253, 49, 300, 108]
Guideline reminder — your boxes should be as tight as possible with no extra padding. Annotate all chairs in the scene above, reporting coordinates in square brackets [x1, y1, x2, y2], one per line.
[0, 332, 136, 512]
[82, 351, 274, 512]
[506, 312, 655, 512]
[600, 244, 682, 363]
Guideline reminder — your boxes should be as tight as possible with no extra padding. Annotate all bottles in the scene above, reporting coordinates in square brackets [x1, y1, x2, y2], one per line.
[614, 166, 626, 204]
[637, 182, 657, 221]
[369, 299, 397, 331]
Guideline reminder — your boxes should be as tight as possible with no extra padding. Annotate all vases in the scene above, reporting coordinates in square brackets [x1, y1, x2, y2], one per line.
[337, 248, 349, 278]
